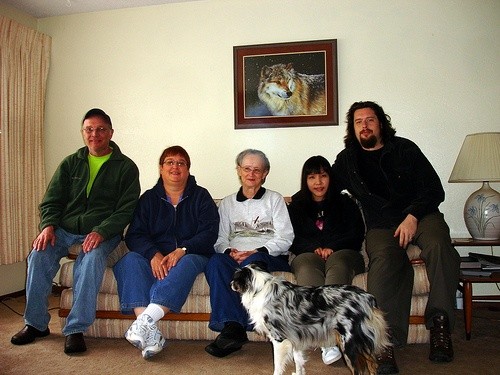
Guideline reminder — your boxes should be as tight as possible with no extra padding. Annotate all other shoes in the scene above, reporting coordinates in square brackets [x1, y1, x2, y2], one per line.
[321, 344, 341, 365]
[205, 325, 250, 358]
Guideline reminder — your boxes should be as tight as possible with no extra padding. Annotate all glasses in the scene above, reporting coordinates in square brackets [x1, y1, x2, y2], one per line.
[239, 163, 266, 174]
[163, 159, 188, 167]
[82, 126, 109, 133]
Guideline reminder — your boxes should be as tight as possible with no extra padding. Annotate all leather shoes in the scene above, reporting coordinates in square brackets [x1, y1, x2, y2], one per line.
[64, 333, 87, 354]
[10, 324, 50, 345]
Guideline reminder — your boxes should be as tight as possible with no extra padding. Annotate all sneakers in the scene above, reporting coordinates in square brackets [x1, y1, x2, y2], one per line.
[427, 314, 454, 362]
[142, 324, 169, 359]
[377, 334, 398, 375]
[125, 313, 154, 350]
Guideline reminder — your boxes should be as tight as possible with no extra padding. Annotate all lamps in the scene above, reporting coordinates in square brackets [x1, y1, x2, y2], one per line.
[448, 132, 500, 240]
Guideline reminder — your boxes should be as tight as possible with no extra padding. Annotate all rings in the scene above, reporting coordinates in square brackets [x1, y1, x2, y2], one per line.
[91, 241, 95, 242]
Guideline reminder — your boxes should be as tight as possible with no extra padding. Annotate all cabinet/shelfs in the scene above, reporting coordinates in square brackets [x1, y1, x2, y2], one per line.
[450, 238, 500, 340]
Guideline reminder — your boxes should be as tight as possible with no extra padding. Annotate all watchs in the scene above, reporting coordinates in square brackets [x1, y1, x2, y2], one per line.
[180, 246, 187, 254]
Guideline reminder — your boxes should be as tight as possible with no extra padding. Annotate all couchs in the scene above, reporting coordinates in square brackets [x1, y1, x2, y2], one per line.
[58, 199, 431, 343]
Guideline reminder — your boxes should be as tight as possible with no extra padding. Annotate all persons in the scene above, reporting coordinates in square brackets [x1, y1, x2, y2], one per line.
[331, 100, 461, 375]
[203, 149, 295, 357]
[10, 108, 141, 355]
[287, 156, 366, 366]
[112, 145, 221, 359]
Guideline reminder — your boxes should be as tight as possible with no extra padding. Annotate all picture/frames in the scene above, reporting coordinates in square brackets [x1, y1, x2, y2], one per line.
[233, 39, 339, 129]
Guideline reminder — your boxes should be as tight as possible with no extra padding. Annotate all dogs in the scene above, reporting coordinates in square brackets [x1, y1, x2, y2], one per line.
[230, 260, 394, 375]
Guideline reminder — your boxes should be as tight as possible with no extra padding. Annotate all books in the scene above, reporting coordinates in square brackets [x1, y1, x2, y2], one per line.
[460, 253, 500, 278]
